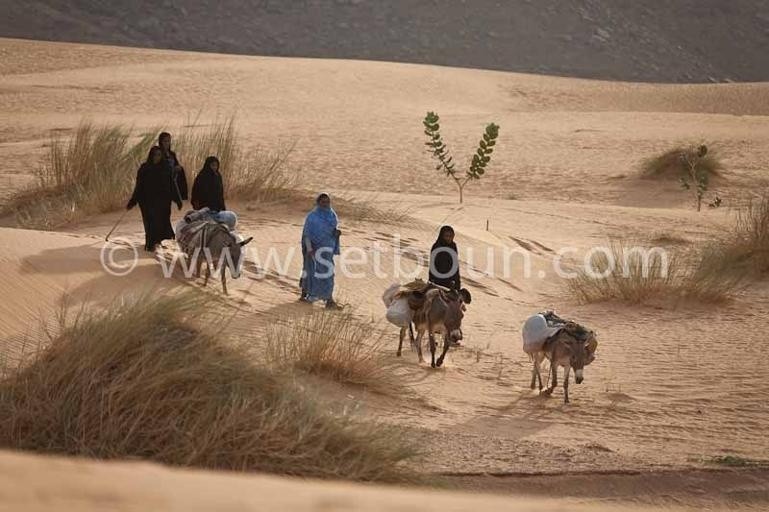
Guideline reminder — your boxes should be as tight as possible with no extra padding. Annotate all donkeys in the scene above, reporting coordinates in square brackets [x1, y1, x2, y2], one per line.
[396, 281, 464, 368]
[530, 316, 590, 403]
[175, 217, 253, 296]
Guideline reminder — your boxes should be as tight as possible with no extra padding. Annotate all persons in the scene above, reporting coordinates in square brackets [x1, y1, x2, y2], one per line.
[157, 131, 188, 202]
[190, 155, 227, 213]
[428, 224, 462, 290]
[125, 145, 183, 253]
[296, 192, 344, 311]
